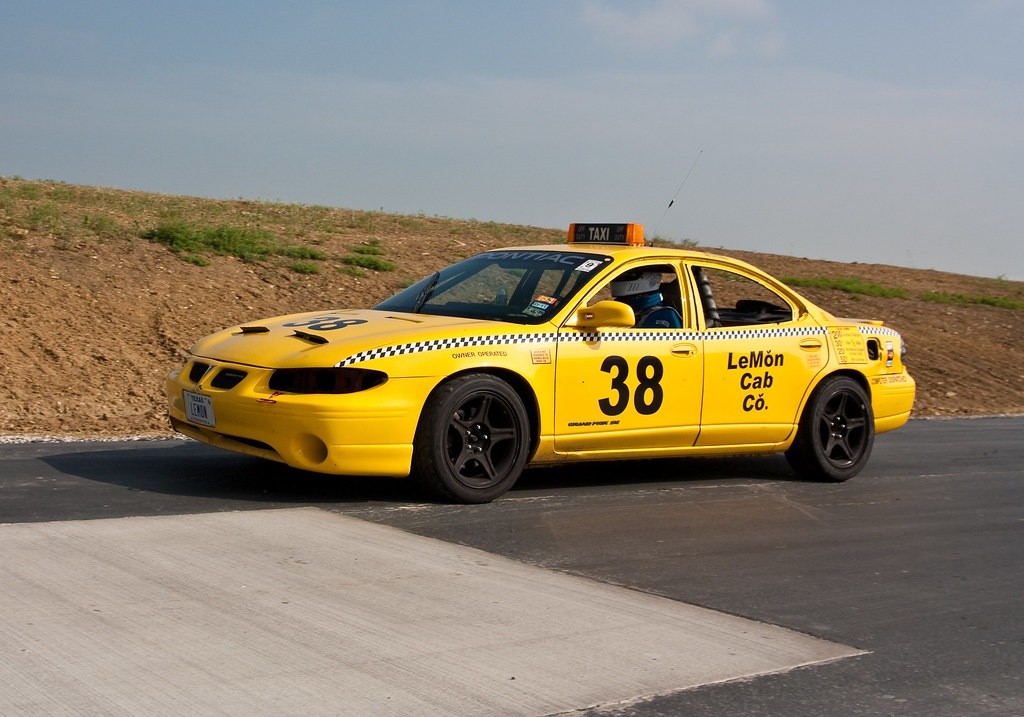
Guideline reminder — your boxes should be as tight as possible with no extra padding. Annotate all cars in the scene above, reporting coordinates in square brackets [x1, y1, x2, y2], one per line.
[161, 221, 918, 504]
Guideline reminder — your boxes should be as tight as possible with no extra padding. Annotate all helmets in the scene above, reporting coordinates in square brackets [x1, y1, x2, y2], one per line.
[610, 272, 662, 297]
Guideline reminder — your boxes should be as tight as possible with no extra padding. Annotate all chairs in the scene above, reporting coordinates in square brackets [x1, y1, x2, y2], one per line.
[658, 278, 718, 328]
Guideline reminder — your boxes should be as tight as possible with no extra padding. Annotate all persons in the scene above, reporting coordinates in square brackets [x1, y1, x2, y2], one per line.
[611, 264, 681, 329]
